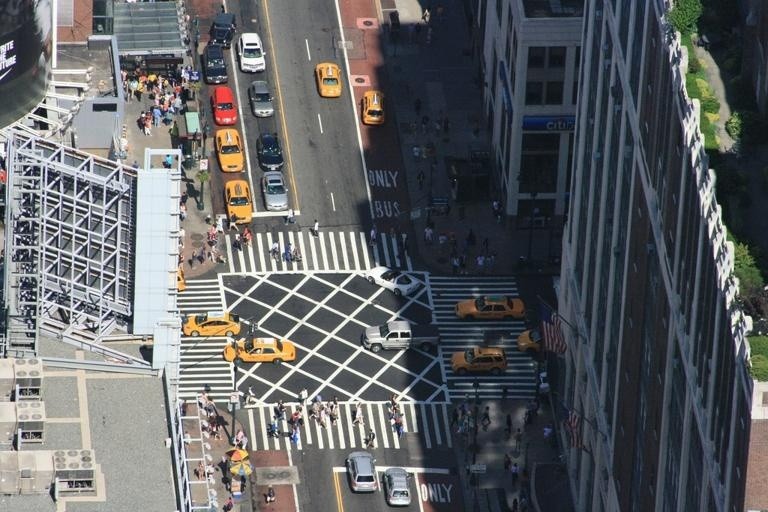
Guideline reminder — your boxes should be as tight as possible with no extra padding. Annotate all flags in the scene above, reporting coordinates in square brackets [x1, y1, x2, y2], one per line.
[566, 410, 582, 448]
[537, 303, 568, 354]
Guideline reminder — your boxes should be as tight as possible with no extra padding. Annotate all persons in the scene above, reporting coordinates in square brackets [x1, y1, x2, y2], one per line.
[164, 154, 173, 169]
[179, 192, 302, 271]
[313, 219, 319, 238]
[371, 222, 497, 275]
[119, 63, 194, 136]
[410, 96, 449, 189]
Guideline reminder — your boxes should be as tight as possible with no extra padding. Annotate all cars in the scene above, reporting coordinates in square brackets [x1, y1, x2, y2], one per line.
[518, 328, 542, 354]
[383, 467, 411, 507]
[224, 338, 295, 365]
[203, 13, 289, 227]
[455, 295, 525, 320]
[361, 90, 386, 125]
[184, 313, 241, 337]
[315, 63, 344, 99]
[451, 348, 507, 376]
[346, 450, 378, 493]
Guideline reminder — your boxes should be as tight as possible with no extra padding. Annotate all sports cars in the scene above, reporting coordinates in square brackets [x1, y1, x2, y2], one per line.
[366, 265, 422, 295]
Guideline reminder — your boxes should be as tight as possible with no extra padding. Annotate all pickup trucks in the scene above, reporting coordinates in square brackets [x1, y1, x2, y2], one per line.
[361, 321, 441, 354]
